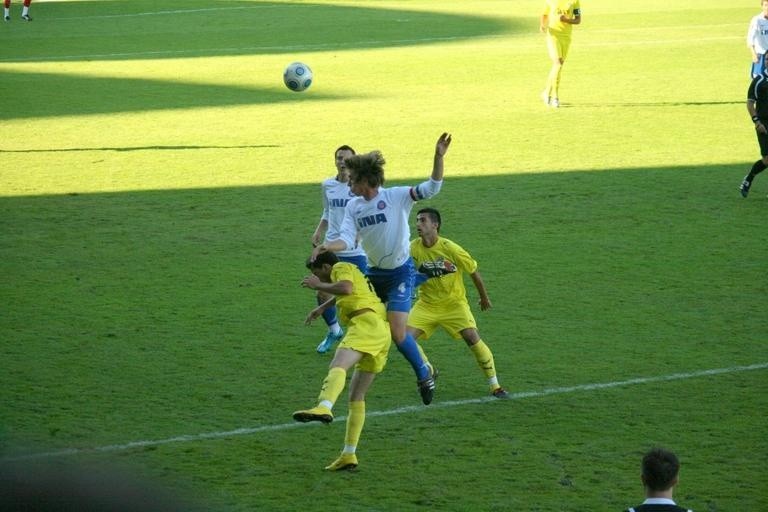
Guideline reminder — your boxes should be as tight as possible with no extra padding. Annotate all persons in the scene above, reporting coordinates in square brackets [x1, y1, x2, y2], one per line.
[4, 0, 32, 22]
[313, 144, 368, 355]
[293, 251, 393, 472]
[310, 133, 452, 405]
[740, 50, 767, 198]
[541, 0, 582, 108]
[625, 450, 695, 512]
[405, 208, 511, 399]
[747, 1, 768, 80]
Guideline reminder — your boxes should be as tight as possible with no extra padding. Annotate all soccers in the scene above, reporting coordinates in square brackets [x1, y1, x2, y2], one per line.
[283, 61, 313, 90]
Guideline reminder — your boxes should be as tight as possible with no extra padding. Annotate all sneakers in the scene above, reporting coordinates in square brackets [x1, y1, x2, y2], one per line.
[292, 404, 334, 425]
[739, 175, 752, 198]
[489, 387, 510, 400]
[324, 451, 358, 471]
[543, 93, 561, 108]
[417, 365, 439, 405]
[4, 14, 33, 23]
[316, 327, 344, 353]
